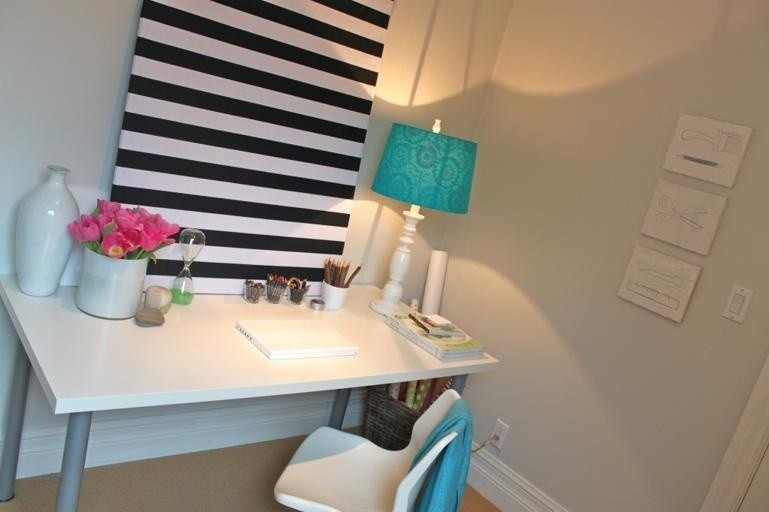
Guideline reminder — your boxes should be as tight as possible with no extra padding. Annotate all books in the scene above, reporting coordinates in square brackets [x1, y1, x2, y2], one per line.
[389, 309, 485, 362]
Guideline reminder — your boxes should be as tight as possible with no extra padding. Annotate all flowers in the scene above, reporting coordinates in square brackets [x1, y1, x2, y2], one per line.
[64, 200, 177, 263]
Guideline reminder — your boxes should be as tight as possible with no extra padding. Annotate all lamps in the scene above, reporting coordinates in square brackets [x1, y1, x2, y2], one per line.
[370, 118, 477, 318]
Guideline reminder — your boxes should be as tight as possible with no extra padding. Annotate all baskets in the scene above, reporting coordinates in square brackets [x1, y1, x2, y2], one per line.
[364, 385, 422, 451]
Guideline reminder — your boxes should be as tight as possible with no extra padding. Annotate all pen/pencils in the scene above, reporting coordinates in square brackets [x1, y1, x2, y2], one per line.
[408, 313, 429, 334]
[264, 272, 289, 304]
[244, 277, 264, 304]
[302, 279, 310, 292]
[324, 256, 363, 288]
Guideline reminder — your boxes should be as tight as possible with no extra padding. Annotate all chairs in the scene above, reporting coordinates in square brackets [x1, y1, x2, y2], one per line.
[270, 391, 470, 511]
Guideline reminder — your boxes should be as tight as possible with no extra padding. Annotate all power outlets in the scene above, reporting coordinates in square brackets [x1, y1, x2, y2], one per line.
[489, 418, 510, 451]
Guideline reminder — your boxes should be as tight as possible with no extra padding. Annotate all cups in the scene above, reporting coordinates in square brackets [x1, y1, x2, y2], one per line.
[241, 284, 264, 305]
[266, 283, 285, 304]
[290, 287, 305, 305]
[322, 279, 351, 311]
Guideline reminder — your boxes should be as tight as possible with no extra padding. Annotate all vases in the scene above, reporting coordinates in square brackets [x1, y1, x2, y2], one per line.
[14, 165, 78, 298]
[75, 245, 150, 321]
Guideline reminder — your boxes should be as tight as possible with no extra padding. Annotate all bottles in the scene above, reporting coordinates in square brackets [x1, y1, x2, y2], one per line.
[410, 298, 420, 312]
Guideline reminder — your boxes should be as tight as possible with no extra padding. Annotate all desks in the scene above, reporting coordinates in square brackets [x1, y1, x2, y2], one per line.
[1, 288, 497, 510]
